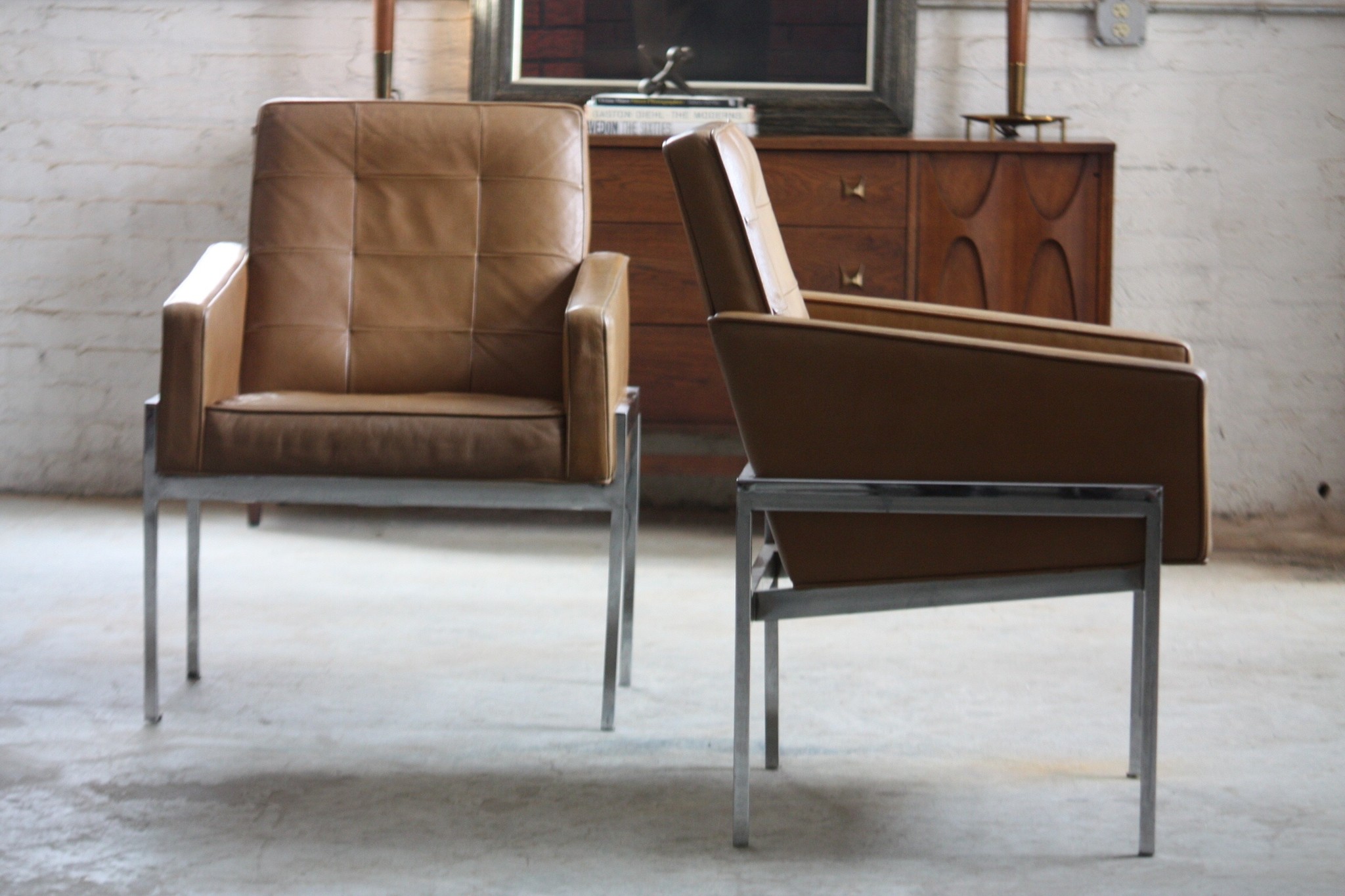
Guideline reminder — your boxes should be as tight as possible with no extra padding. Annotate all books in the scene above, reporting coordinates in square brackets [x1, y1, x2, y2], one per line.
[582, 94, 758, 138]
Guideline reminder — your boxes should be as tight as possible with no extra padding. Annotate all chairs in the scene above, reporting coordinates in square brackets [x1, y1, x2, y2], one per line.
[143, 97, 642, 734]
[661, 119, 1213, 858]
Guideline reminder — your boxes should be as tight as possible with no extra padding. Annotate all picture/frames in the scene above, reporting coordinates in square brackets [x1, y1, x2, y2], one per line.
[468, 1, 917, 136]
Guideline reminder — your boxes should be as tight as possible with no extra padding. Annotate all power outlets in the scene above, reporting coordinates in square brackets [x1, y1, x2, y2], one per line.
[1094, 0, 1146, 46]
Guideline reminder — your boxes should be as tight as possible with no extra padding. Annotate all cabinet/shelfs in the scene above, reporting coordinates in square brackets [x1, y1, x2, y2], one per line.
[589, 136, 1117, 476]
